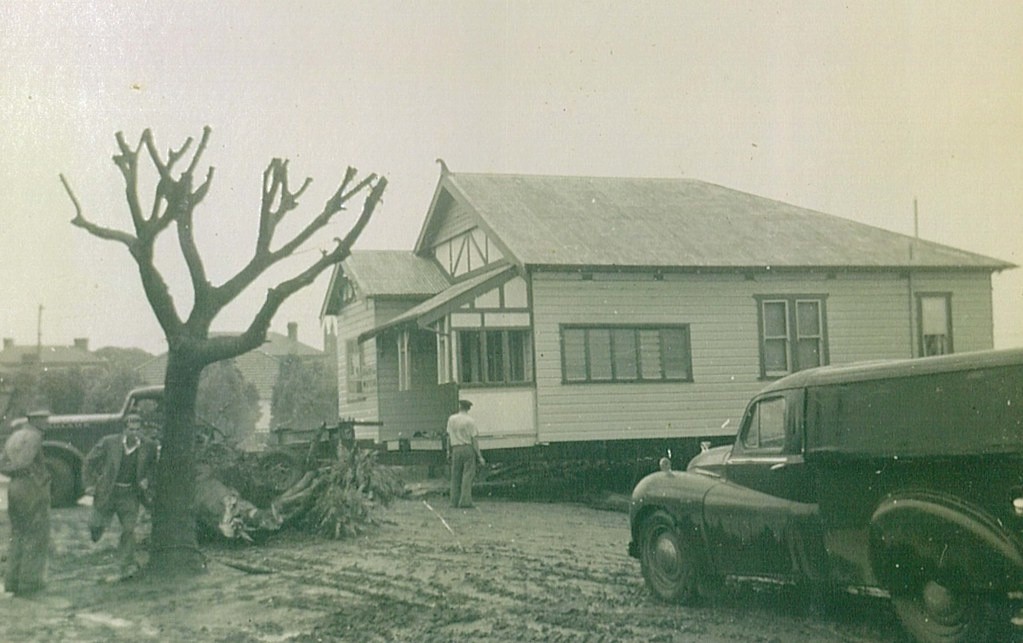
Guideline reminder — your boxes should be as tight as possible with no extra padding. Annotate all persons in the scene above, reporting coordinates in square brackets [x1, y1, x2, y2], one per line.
[445, 399, 486, 507]
[4, 410, 51, 598]
[82, 415, 147, 566]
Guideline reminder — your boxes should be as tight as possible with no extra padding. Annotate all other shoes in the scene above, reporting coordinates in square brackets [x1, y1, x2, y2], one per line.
[92, 529, 102, 543]
[459, 504, 476, 508]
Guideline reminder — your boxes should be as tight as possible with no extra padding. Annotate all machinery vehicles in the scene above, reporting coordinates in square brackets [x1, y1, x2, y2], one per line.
[7, 385, 304, 510]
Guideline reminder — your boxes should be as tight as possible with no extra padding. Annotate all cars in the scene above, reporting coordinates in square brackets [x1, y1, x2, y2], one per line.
[626, 347, 1023, 642]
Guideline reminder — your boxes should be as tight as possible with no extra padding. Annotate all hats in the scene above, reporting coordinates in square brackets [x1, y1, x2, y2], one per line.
[25, 411, 51, 421]
[459, 401, 473, 408]
